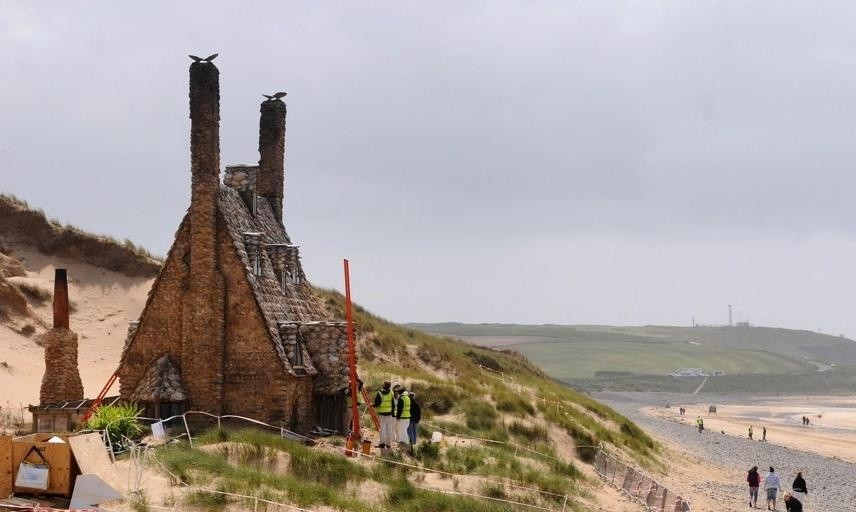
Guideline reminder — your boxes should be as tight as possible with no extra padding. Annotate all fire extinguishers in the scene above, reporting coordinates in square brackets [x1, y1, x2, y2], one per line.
[346, 430, 353, 456]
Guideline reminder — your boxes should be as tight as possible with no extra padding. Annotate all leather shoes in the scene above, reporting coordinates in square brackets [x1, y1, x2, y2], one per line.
[374, 444, 390, 448]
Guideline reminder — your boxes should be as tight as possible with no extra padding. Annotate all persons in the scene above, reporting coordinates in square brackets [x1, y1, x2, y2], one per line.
[748, 425, 753, 440]
[782, 493, 802, 512]
[763, 466, 782, 510]
[761, 426, 766, 442]
[341, 378, 364, 437]
[746, 466, 762, 509]
[370, 379, 421, 449]
[792, 471, 808, 511]
[696, 415, 703, 433]
[679, 406, 685, 415]
[802, 415, 809, 425]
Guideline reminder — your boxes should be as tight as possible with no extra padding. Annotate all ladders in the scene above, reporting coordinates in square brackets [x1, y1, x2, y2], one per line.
[360, 383, 381, 431]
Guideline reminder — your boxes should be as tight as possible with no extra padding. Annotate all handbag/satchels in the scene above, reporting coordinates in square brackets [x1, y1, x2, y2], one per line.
[14, 445, 50, 491]
